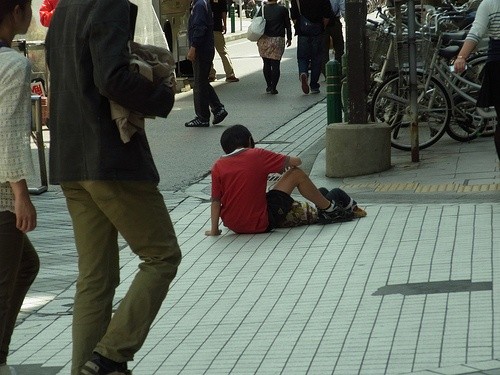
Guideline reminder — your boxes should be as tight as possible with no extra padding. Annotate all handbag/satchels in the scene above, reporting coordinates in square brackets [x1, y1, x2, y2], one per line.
[299, 16, 323, 36]
[247, 3, 266, 41]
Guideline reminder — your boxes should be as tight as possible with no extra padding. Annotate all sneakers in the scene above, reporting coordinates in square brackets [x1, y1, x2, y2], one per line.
[318, 205, 354, 224]
[213, 109, 229, 124]
[185, 117, 207, 127]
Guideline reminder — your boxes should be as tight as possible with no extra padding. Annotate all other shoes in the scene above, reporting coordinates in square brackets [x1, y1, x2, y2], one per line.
[307, 67, 311, 73]
[311, 88, 320, 94]
[300, 72, 309, 93]
[271, 87, 279, 94]
[208, 76, 215, 82]
[81, 352, 131, 375]
[266, 85, 272, 92]
[226, 77, 239, 82]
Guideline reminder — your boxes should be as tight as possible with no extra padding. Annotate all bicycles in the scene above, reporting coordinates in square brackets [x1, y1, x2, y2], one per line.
[338, 0, 498, 151]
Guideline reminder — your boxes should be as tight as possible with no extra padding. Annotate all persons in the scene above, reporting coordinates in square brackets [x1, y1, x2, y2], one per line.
[0, 0, 40, 375]
[40, 0, 60, 27]
[45, 0, 181, 375]
[205, 124, 353, 236]
[208, 0, 346, 94]
[455, 0, 500, 160]
[185, 0, 228, 127]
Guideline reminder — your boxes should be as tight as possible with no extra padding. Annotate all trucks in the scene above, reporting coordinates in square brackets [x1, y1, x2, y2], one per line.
[11, 0, 194, 129]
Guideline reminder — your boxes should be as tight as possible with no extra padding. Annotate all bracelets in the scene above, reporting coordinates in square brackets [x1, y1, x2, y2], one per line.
[458, 56, 465, 60]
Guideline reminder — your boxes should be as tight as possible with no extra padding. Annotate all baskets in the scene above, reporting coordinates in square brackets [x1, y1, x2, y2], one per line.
[364, 24, 391, 63]
[398, 39, 424, 62]
